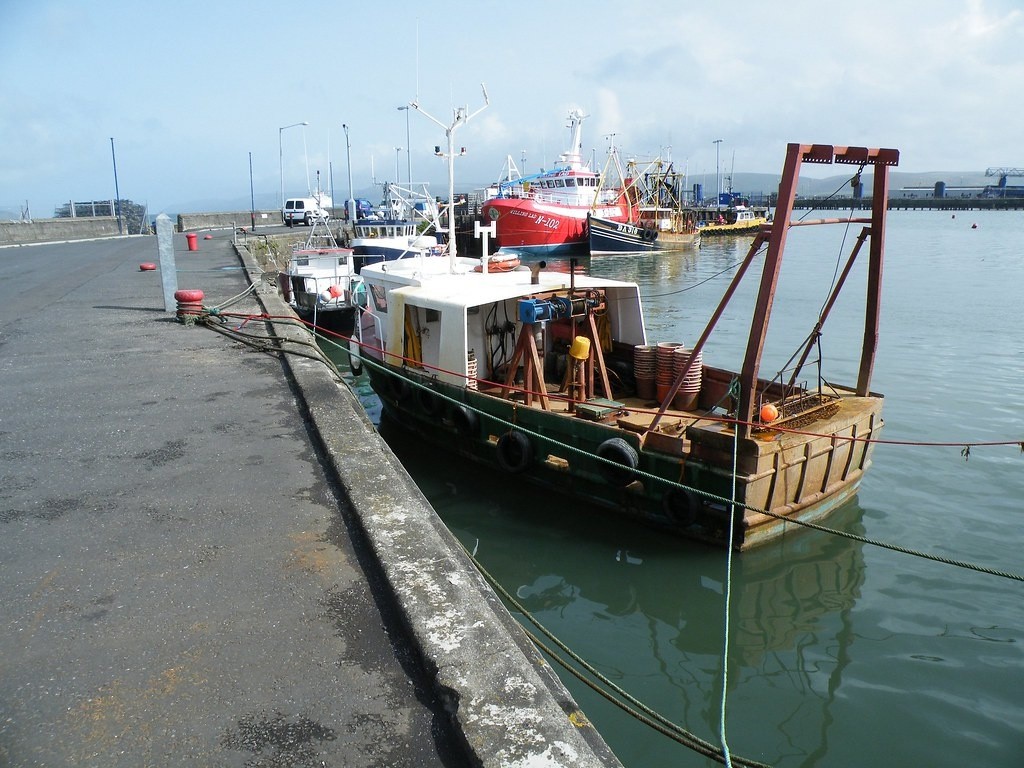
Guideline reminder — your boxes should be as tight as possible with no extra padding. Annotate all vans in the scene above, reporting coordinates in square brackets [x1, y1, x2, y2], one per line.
[283, 197, 329, 225]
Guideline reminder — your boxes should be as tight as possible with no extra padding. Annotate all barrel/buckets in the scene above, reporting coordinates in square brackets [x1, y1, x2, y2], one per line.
[634, 341, 703, 411]
[569, 336, 591, 359]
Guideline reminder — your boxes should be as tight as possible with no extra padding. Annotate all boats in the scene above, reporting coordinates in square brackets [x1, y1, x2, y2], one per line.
[277, 109, 772, 327]
[350, 138, 903, 555]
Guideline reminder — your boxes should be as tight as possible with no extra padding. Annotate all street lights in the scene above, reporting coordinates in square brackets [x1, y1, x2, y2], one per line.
[713, 140, 723, 218]
[398, 106, 412, 199]
[278, 122, 308, 223]
[396, 145, 404, 201]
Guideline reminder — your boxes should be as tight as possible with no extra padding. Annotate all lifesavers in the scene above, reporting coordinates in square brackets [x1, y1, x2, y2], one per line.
[701, 225, 760, 237]
[473, 252, 520, 273]
[389, 374, 411, 403]
[416, 383, 442, 417]
[594, 436, 640, 489]
[451, 404, 479, 439]
[495, 431, 532, 475]
[651, 230, 659, 241]
[661, 483, 699, 529]
[642, 228, 652, 240]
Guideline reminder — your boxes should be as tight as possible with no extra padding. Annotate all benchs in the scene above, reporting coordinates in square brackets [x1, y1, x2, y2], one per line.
[294, 291, 326, 310]
